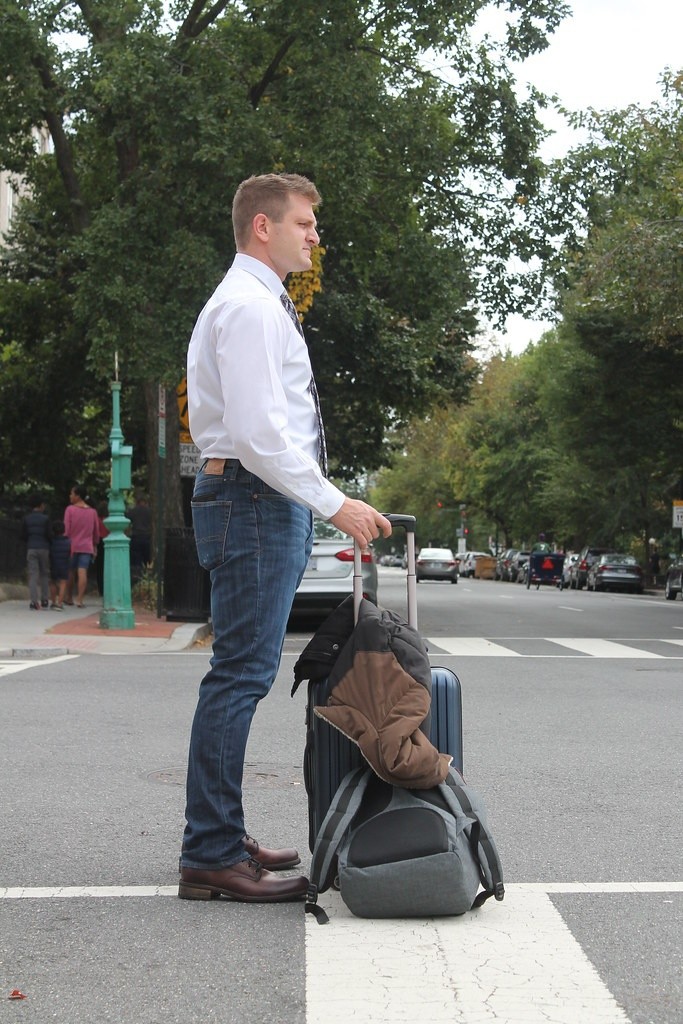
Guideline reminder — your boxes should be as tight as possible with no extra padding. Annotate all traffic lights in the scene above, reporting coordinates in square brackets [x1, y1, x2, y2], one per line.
[437, 502, 446, 509]
[463, 523, 470, 538]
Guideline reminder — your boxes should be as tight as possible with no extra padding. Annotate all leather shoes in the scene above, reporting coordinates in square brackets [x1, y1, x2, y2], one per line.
[240, 831, 300, 868]
[177, 855, 311, 906]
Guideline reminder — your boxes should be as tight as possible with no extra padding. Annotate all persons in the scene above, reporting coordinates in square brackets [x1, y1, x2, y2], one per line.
[95, 501, 109, 596]
[127, 499, 153, 590]
[63, 485, 99, 608]
[531, 533, 551, 553]
[45, 520, 70, 611]
[649, 546, 660, 581]
[19, 493, 51, 610]
[178, 173, 391, 903]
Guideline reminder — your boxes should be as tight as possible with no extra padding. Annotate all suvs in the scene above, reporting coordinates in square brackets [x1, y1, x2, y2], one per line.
[560, 543, 618, 590]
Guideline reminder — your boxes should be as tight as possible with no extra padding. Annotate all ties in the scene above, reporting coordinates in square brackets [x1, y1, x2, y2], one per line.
[282, 290, 329, 485]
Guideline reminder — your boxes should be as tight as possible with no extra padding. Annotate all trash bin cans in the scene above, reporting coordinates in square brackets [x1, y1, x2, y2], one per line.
[163, 527, 210, 624]
[474, 557, 497, 580]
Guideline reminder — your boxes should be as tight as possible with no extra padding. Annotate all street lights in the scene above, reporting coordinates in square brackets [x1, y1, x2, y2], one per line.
[648, 538, 656, 556]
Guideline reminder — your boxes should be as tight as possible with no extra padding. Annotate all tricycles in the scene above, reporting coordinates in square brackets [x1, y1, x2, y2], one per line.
[518, 551, 566, 592]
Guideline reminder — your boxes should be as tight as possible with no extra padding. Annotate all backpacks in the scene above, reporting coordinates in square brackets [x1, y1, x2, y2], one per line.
[303, 746, 505, 921]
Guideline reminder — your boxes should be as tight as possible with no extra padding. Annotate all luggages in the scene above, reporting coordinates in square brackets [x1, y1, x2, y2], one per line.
[302, 510, 464, 852]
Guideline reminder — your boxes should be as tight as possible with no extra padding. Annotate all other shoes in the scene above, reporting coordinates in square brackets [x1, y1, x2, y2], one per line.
[77, 602, 85, 608]
[41, 599, 49, 607]
[50, 603, 64, 612]
[64, 599, 73, 605]
[30, 601, 37, 611]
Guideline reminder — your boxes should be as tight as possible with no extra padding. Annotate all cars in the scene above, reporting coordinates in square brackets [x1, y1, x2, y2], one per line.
[586, 553, 645, 594]
[456, 551, 491, 578]
[415, 547, 460, 584]
[497, 546, 531, 585]
[287, 514, 379, 623]
[381, 553, 410, 569]
[664, 551, 683, 601]
